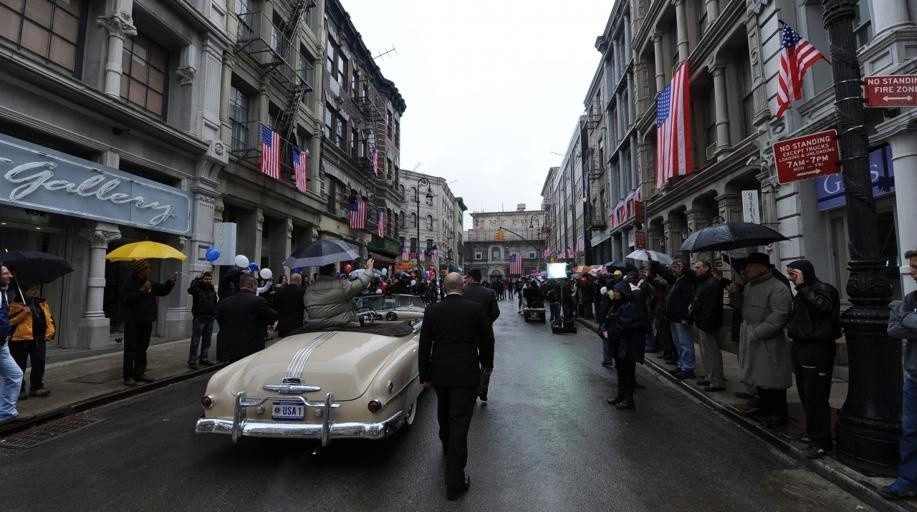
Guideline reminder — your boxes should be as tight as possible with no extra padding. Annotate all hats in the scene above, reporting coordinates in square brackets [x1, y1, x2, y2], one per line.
[741, 252, 775, 267]
[613, 269, 622, 278]
[627, 272, 638, 279]
[135, 261, 150, 273]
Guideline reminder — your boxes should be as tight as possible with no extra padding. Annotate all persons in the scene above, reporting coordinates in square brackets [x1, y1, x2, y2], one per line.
[7, 278, 57, 401]
[118, 260, 178, 387]
[188, 258, 442, 369]
[784, 258, 842, 458]
[0, 263, 24, 425]
[464, 272, 500, 406]
[514, 262, 743, 409]
[738, 252, 793, 428]
[482, 274, 521, 299]
[876, 251, 916, 500]
[416, 271, 487, 500]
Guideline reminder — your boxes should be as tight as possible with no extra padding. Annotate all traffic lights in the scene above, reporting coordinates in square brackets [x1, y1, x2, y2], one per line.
[501, 231, 504, 240]
[496, 231, 500, 240]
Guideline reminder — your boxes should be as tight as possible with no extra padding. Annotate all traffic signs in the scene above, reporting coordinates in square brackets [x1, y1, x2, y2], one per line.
[773, 130, 840, 184]
[864, 77, 917, 107]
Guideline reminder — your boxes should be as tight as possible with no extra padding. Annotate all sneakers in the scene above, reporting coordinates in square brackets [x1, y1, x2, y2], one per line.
[29, 388, 50, 396]
[137, 374, 155, 382]
[877, 476, 916, 500]
[199, 360, 216, 366]
[189, 363, 201, 369]
[806, 447, 833, 458]
[0, 408, 34, 427]
[801, 436, 814, 442]
[18, 392, 28, 400]
[669, 368, 726, 391]
[124, 379, 135, 386]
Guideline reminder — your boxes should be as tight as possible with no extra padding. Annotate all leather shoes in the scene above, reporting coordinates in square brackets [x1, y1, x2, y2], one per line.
[446, 474, 470, 501]
[735, 390, 779, 424]
[616, 401, 635, 409]
[607, 398, 620, 404]
[602, 360, 612, 366]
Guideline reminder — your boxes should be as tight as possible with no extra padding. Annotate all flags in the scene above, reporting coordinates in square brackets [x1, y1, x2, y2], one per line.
[368, 142, 378, 176]
[653, 61, 692, 189]
[774, 21, 824, 122]
[291, 144, 307, 194]
[348, 198, 366, 231]
[259, 125, 281, 182]
[509, 253, 521, 273]
[543, 245, 551, 259]
[566, 246, 575, 259]
[375, 208, 384, 239]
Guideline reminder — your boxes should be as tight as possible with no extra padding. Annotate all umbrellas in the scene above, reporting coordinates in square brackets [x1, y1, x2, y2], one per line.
[281, 238, 361, 271]
[675, 221, 790, 285]
[0, 250, 74, 305]
[101, 240, 188, 283]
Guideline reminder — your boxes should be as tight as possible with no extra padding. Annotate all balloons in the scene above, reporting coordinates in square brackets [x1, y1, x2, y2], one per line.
[235, 255, 272, 279]
[206, 249, 220, 260]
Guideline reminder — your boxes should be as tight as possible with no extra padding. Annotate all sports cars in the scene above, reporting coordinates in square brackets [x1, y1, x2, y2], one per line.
[195, 293, 436, 452]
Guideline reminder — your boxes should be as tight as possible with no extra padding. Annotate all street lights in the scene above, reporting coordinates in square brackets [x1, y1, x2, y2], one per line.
[530, 214, 541, 273]
[416, 175, 435, 290]
